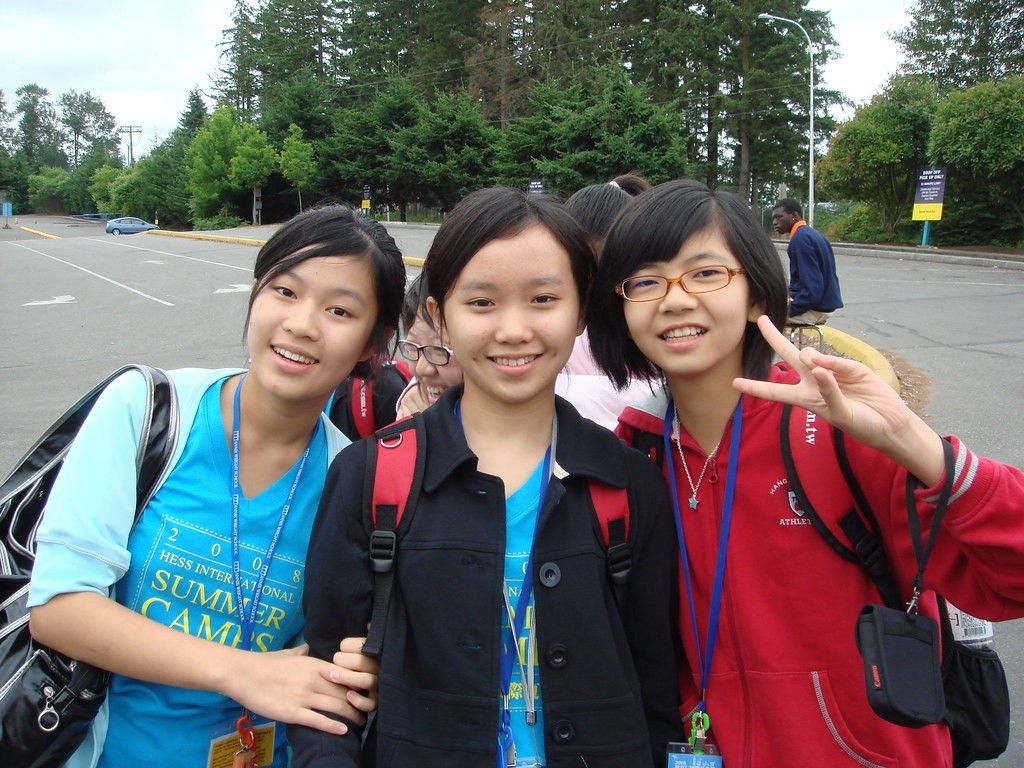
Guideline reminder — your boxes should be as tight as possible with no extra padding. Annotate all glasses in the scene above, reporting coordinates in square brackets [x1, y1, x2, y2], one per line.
[613, 264, 747, 303]
[397, 339, 453, 367]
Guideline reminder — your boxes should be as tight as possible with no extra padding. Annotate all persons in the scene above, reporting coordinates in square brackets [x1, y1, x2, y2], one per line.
[386, 174, 931, 431]
[284, 183, 683, 768]
[26, 210, 406, 767]
[587, 178, 1023, 768]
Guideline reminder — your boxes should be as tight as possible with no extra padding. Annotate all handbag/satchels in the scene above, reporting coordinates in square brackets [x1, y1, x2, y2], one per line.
[854, 604, 946, 730]
[0, 362, 180, 768]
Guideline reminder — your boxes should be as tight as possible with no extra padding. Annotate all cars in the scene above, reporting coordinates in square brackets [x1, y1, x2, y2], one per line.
[105, 216, 161, 237]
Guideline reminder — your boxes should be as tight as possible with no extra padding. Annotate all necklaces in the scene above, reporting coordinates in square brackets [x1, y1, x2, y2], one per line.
[668, 405, 719, 512]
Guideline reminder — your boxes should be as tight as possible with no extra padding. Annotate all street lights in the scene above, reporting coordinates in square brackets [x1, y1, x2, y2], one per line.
[759, 13, 814, 229]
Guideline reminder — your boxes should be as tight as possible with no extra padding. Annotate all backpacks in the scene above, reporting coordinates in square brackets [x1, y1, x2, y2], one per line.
[628, 377, 1012, 768]
[329, 353, 414, 443]
